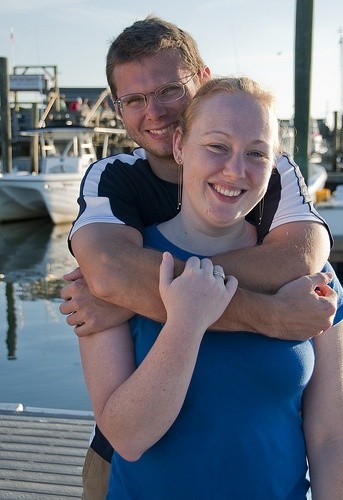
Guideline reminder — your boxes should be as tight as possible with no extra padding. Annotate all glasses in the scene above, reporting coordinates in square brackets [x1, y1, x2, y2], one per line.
[111, 70, 199, 115]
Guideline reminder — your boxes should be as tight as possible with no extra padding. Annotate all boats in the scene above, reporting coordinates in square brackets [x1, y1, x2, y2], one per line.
[1, 125, 104, 224]
[306, 161, 343, 262]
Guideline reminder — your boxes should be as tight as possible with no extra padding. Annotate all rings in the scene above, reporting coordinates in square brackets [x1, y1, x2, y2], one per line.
[213, 270, 225, 281]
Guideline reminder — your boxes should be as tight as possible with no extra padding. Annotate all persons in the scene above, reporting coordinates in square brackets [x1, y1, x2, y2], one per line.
[59, 15, 338, 499]
[68, 76, 343, 499]
[57, 93, 116, 128]
